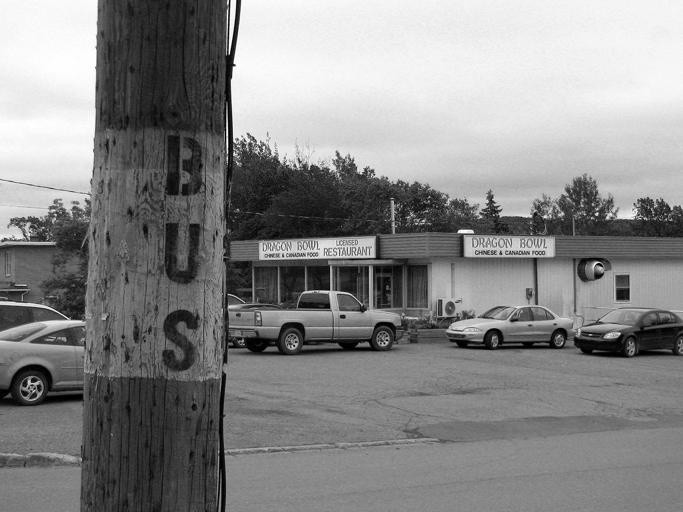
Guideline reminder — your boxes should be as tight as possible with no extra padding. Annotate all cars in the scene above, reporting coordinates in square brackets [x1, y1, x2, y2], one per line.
[0, 320, 87, 406]
[573, 306, 683, 357]
[0, 299, 70, 332]
[446, 304, 573, 350]
[225, 303, 285, 349]
[226, 292, 247, 306]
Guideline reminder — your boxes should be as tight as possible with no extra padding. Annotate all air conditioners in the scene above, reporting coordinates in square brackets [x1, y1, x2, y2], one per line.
[436, 298, 462, 319]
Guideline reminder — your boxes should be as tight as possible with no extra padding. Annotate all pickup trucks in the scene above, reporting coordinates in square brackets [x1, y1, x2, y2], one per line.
[228, 289, 402, 355]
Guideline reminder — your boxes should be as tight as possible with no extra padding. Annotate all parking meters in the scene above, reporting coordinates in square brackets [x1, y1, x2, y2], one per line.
[525, 286, 533, 305]
